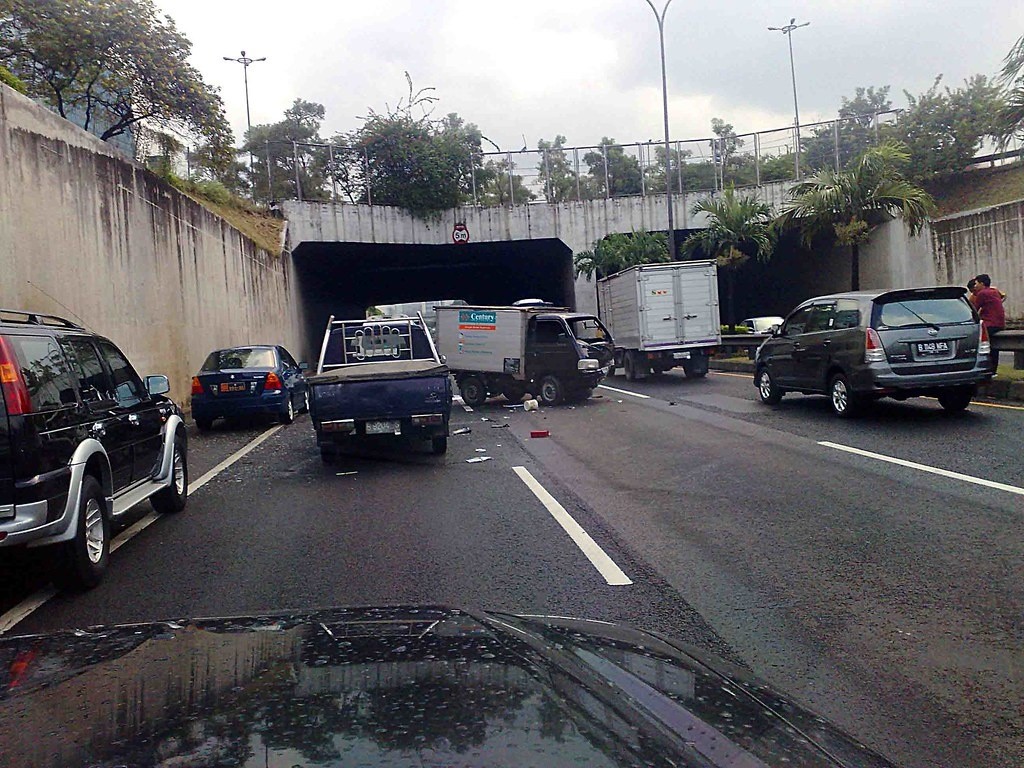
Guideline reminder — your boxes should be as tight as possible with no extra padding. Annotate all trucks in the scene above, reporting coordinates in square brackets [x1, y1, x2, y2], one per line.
[433, 299, 617, 406]
[306, 310, 454, 464]
[597, 258, 722, 384]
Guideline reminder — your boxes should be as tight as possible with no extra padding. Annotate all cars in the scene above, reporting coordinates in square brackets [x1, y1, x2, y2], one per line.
[740, 317, 785, 335]
[752, 286, 993, 419]
[191, 344, 310, 431]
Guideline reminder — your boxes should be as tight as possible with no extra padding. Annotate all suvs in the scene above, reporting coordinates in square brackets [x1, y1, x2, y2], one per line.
[0, 310, 190, 594]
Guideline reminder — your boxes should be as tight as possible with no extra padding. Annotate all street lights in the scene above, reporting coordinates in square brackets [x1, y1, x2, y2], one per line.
[768, 17, 811, 159]
[223, 51, 267, 201]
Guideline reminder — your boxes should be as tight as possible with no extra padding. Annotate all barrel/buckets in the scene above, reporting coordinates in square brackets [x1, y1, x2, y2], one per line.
[524, 399, 538, 411]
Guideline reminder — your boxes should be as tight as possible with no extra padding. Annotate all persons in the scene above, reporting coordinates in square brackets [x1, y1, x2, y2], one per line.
[967, 279, 1008, 313]
[971, 274, 1006, 377]
